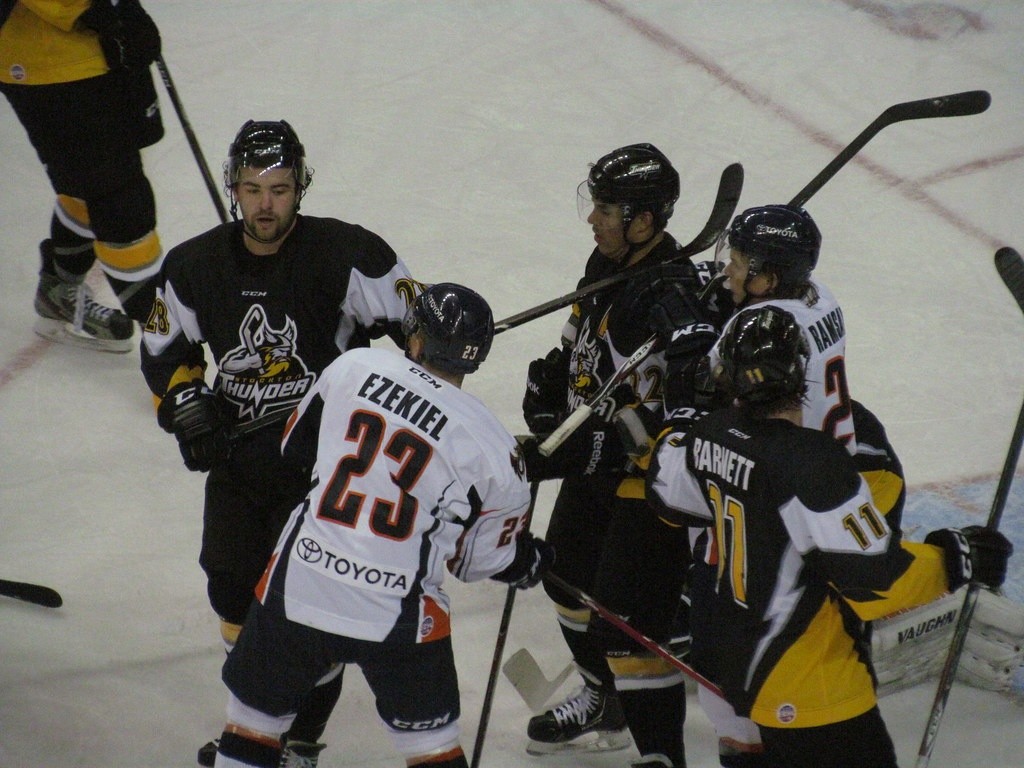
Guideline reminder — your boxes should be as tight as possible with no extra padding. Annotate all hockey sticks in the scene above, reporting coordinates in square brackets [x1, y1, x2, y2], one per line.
[0, 579, 63, 607]
[914, 247, 1024, 768]
[235, 162, 745, 437]
[538, 90, 992, 458]
[536, 564, 767, 768]
[154, 53, 227, 224]
[469, 436, 539, 768]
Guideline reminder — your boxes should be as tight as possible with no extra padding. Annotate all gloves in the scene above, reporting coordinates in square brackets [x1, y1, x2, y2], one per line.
[523, 414, 625, 482]
[490, 526, 556, 591]
[91, 0, 161, 77]
[663, 351, 726, 413]
[521, 356, 566, 434]
[922, 524, 1015, 593]
[157, 379, 237, 473]
[629, 272, 718, 359]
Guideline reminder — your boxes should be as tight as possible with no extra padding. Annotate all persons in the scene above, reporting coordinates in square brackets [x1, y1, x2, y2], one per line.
[1, 0, 164, 352]
[709, 204, 907, 669]
[647, 305, 1014, 768]
[140, 120, 435, 767]
[523, 144, 703, 768]
[213, 286, 559, 768]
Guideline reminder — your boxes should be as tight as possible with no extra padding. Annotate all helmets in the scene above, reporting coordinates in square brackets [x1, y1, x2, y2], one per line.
[228, 119, 307, 194]
[724, 303, 800, 397]
[416, 281, 496, 374]
[728, 203, 822, 293]
[587, 141, 679, 229]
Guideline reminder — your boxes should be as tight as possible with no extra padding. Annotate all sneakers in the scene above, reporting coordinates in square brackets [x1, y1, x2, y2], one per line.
[35, 239, 134, 352]
[524, 660, 631, 756]
[279, 738, 327, 768]
[197, 737, 222, 767]
